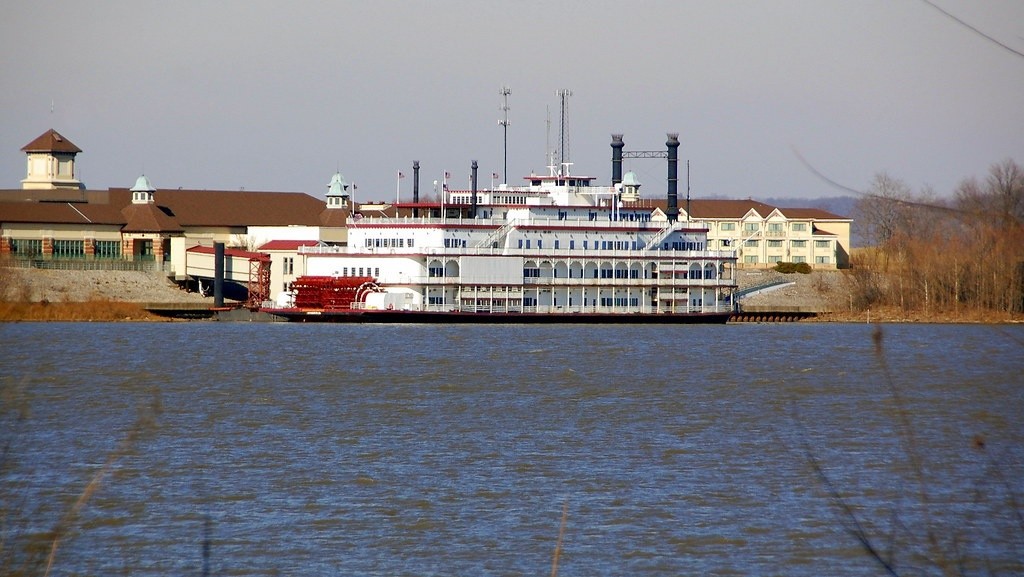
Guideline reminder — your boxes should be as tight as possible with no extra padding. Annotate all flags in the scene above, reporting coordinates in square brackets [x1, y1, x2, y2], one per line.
[470, 175, 473, 181]
[493, 173, 499, 179]
[399, 172, 405, 178]
[354, 185, 358, 189]
[445, 171, 451, 178]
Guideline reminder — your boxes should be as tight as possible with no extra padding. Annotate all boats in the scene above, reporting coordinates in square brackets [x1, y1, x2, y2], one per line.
[241, 152, 796, 325]
